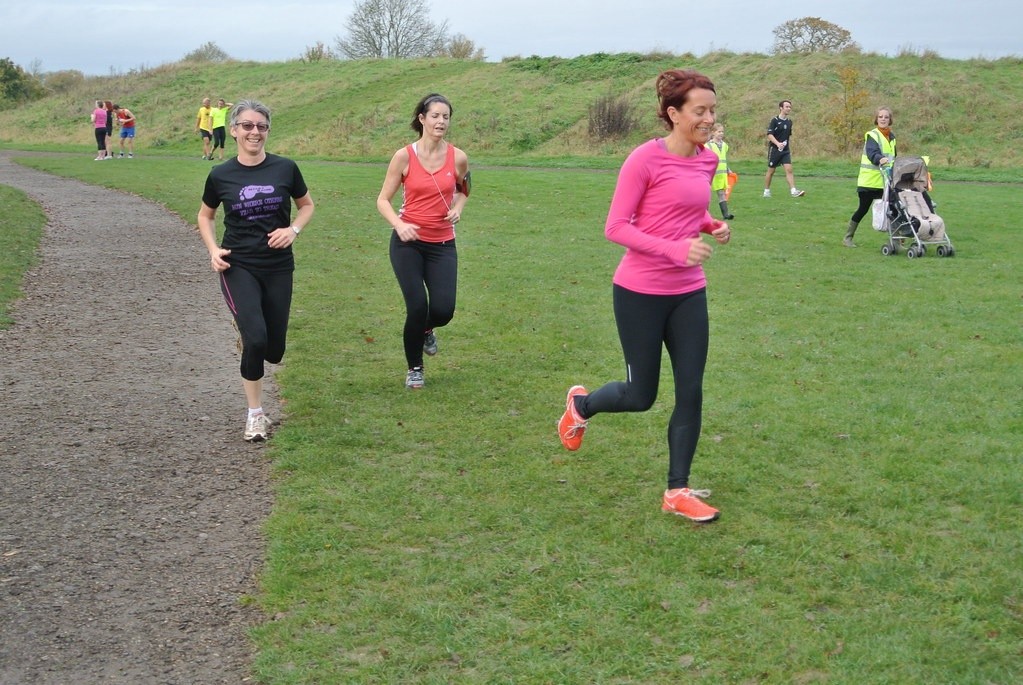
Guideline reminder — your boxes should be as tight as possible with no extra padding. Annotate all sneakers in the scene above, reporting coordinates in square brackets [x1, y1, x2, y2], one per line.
[424, 327, 438, 355]
[791, 189, 804, 198]
[94, 156, 105, 160]
[558, 385, 588, 452]
[661, 487, 720, 522]
[762, 192, 771, 198]
[406, 364, 425, 389]
[229, 319, 244, 356]
[244, 412, 268, 441]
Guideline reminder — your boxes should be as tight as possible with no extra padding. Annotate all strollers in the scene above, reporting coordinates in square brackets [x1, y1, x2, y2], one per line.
[878, 156, 956, 259]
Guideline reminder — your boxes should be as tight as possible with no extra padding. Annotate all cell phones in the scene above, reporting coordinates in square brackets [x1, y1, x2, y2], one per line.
[465, 174, 471, 194]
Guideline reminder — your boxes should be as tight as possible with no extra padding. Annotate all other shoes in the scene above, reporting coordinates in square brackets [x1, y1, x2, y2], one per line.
[210, 157, 214, 160]
[218, 158, 223, 160]
[127, 153, 132, 158]
[105, 156, 113, 160]
[202, 155, 210, 160]
[117, 153, 123, 158]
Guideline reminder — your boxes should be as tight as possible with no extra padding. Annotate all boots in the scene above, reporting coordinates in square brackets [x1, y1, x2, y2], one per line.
[718, 200, 734, 220]
[843, 220, 858, 249]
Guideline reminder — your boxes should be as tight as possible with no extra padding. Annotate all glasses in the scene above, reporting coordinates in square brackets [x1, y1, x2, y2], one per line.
[236, 119, 270, 132]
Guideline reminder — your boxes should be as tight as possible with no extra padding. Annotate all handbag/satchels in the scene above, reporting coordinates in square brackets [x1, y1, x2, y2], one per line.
[872, 185, 890, 232]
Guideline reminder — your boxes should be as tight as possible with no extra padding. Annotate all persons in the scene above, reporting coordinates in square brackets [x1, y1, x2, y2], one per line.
[763, 100, 804, 198]
[198, 99, 314, 442]
[703, 123, 734, 220]
[377, 93, 472, 387]
[843, 106, 898, 248]
[195, 98, 234, 161]
[90, 100, 136, 161]
[557, 69, 730, 521]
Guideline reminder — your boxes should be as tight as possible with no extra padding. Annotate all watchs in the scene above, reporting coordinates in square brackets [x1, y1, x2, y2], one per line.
[290, 225, 300, 235]
